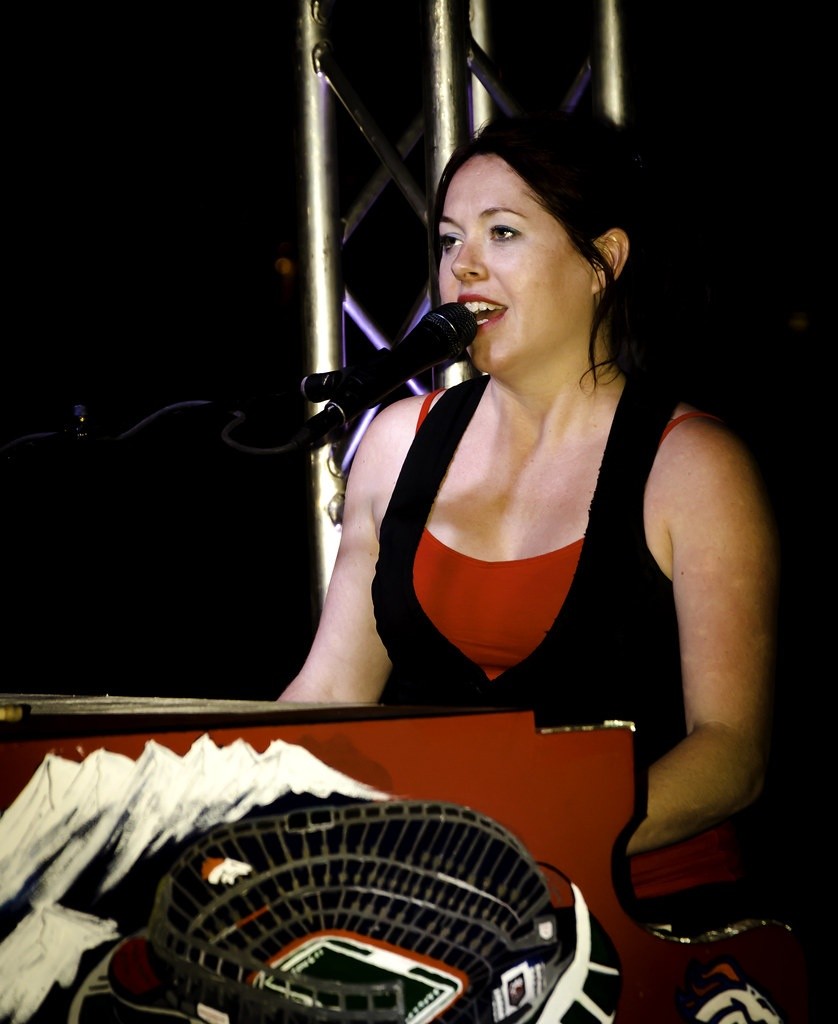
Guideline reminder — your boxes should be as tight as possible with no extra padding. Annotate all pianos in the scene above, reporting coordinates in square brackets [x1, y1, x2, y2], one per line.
[0, 686, 815, 1024]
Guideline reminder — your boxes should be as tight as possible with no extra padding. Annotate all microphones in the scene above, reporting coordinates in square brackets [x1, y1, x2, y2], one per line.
[289, 302, 478, 453]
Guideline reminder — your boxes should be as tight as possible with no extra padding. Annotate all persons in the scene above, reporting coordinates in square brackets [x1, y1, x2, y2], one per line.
[276, 110, 776, 934]
